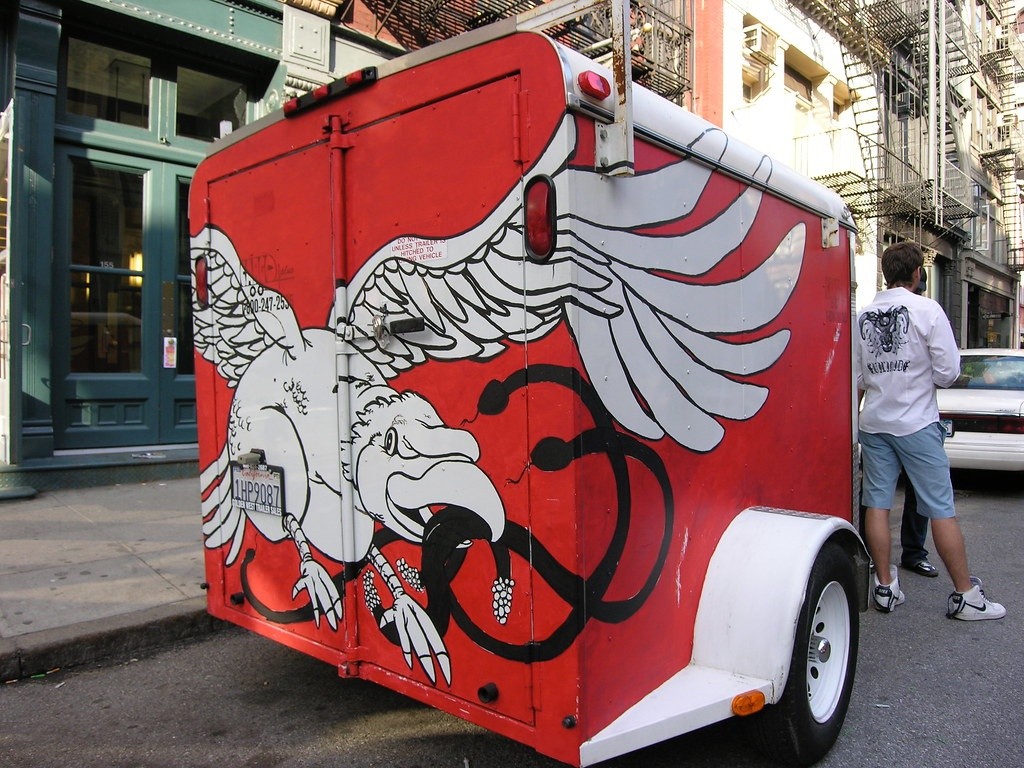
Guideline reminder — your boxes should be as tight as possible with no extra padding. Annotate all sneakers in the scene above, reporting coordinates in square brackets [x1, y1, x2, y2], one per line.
[905, 559, 939, 578]
[873, 565, 905, 613]
[949, 575, 1007, 621]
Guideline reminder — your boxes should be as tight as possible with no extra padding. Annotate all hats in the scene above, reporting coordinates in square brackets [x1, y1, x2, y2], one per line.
[918, 268, 926, 291]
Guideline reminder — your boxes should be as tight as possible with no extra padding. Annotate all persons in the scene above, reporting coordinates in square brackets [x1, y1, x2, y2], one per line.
[856, 240, 1007, 621]
[856, 267, 939, 577]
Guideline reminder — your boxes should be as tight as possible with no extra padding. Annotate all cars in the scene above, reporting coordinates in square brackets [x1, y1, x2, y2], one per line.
[934, 348, 1024, 472]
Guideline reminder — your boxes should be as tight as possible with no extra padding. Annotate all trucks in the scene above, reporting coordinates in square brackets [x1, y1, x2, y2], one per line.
[189, 28, 873, 768]
